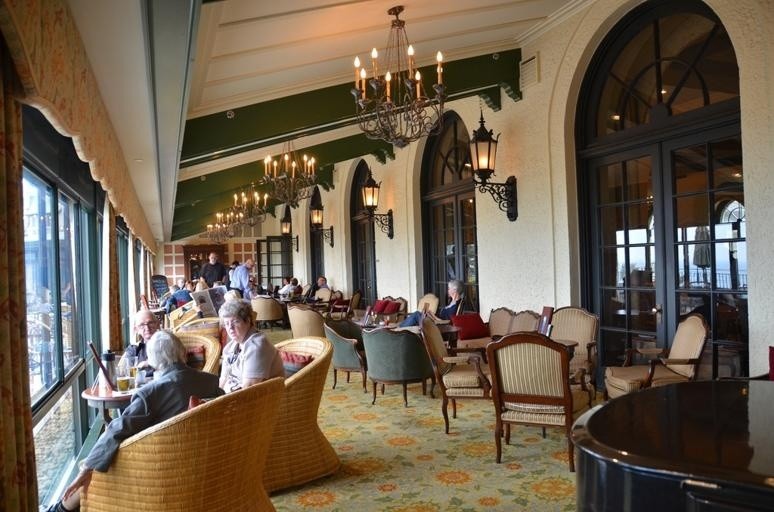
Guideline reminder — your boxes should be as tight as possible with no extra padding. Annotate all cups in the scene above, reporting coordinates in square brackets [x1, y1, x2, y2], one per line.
[99, 352, 146, 397]
[288, 290, 294, 300]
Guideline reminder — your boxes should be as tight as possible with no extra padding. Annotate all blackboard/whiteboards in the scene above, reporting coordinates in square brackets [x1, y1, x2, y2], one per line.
[151, 275, 169, 299]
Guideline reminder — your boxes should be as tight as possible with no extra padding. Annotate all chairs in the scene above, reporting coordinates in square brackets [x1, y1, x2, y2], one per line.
[602, 313, 710, 402]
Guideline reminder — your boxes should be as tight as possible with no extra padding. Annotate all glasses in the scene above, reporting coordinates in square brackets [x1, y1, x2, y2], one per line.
[222, 318, 245, 327]
[134, 319, 156, 329]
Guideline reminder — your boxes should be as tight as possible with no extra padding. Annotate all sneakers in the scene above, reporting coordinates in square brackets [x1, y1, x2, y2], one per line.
[37, 502, 53, 512]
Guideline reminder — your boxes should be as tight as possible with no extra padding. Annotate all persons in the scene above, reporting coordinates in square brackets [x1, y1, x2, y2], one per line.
[160, 251, 330, 321]
[218, 297, 285, 394]
[37, 330, 221, 512]
[117, 310, 188, 376]
[398, 279, 465, 328]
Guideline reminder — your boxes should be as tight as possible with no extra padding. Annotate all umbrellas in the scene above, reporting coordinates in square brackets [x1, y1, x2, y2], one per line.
[692, 226, 711, 268]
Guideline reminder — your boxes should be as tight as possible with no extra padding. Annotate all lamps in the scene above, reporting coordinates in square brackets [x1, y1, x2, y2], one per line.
[263, 141, 315, 208]
[309, 200, 334, 248]
[351, 7, 447, 148]
[361, 165, 393, 239]
[469, 110, 518, 222]
[280, 215, 298, 252]
[206, 181, 269, 245]
